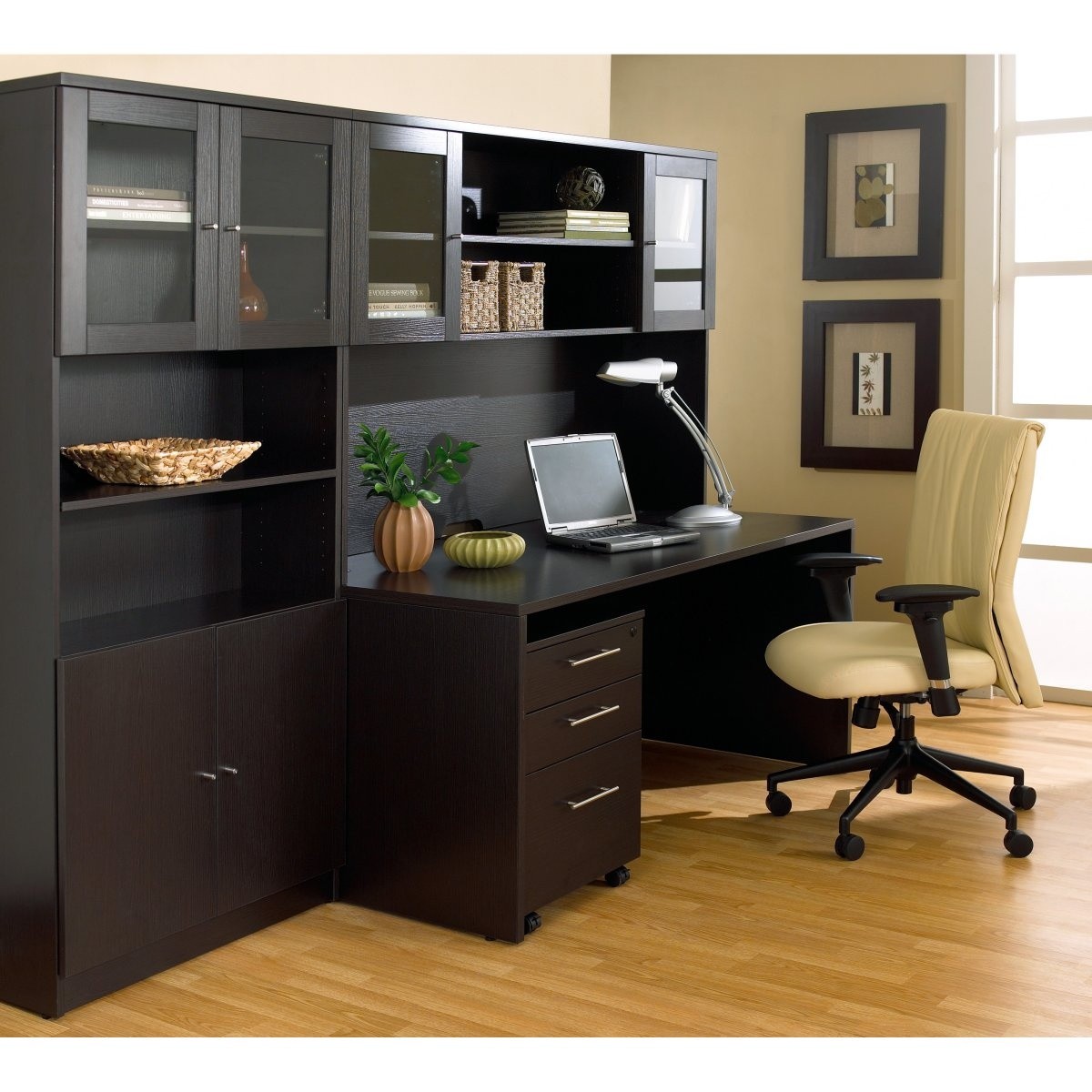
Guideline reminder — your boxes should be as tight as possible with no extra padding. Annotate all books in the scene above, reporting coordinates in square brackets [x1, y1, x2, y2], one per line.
[495, 207, 633, 242]
[83, 180, 198, 226]
[657, 173, 703, 242]
[363, 276, 444, 319]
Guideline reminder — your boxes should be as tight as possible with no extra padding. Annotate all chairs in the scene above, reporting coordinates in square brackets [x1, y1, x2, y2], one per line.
[760, 402, 1044, 867]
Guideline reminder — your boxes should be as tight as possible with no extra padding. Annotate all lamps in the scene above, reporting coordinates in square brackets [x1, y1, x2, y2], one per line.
[595, 357, 741, 530]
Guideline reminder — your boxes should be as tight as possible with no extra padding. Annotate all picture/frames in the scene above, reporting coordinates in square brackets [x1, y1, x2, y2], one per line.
[797, 102, 945, 280]
[797, 299, 938, 475]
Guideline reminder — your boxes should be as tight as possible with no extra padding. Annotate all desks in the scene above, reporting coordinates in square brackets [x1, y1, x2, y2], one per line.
[342, 502, 860, 955]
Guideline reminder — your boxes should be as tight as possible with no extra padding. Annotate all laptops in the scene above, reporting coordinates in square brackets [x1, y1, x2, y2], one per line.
[524, 432, 701, 555]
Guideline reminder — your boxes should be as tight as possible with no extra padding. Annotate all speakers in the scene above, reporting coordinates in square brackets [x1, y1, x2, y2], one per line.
[462, 188, 482, 234]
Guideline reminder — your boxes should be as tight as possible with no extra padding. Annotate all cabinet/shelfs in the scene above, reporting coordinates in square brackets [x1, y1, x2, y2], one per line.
[515, 608, 645, 936]
[1, 72, 356, 1019]
[355, 106, 716, 340]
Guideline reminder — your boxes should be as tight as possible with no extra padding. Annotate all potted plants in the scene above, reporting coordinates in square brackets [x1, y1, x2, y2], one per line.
[351, 425, 482, 571]
